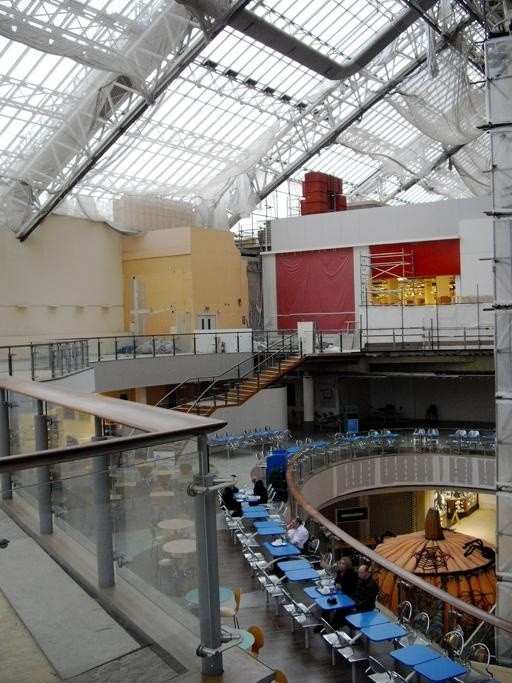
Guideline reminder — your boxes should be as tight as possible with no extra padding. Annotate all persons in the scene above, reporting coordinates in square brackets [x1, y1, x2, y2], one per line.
[321, 564, 379, 635]
[313, 556, 356, 633]
[223, 475, 244, 517]
[271, 518, 310, 572]
[249, 476, 268, 505]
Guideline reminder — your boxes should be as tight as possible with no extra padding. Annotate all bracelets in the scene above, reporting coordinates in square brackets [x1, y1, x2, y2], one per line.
[286, 527, 289, 530]
